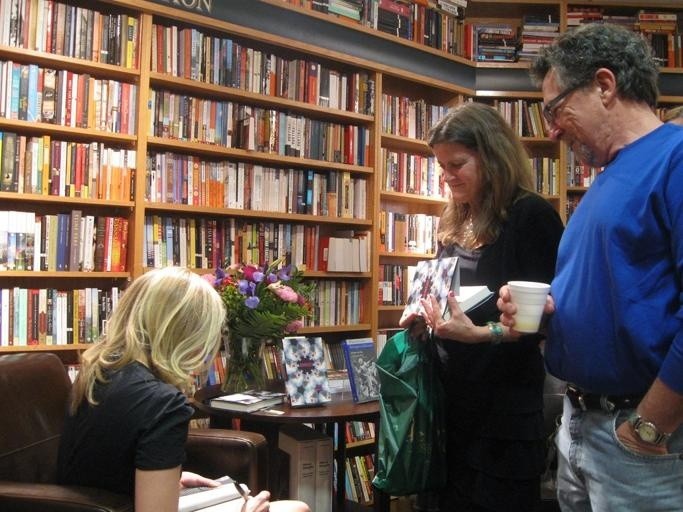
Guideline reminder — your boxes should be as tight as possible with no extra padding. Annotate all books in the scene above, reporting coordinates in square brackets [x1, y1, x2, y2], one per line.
[177, 472, 248, 512]
[1, 1, 138, 70]
[187, 415, 212, 432]
[2, 286, 125, 345]
[189, 331, 389, 408]
[2, 130, 136, 201]
[378, 2, 682, 327]
[276, 418, 378, 512]
[62, 362, 84, 385]
[2, 60, 136, 135]
[1, 209, 129, 272]
[145, 1, 378, 326]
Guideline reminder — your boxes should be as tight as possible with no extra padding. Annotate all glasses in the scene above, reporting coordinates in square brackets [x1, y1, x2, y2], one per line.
[543, 86, 575, 122]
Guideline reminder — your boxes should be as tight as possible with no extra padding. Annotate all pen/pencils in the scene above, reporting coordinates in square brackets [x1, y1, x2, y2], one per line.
[233, 481, 249, 502]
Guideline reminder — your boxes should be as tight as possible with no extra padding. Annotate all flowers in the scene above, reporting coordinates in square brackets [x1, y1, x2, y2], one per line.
[200, 255, 318, 388]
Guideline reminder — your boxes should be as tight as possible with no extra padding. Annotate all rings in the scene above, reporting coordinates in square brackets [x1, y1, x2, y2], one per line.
[425, 328, 433, 340]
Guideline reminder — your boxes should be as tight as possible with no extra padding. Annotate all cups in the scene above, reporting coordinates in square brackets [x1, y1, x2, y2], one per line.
[507, 280, 550, 335]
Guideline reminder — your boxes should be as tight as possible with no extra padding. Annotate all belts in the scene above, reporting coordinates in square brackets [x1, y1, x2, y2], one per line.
[566, 386, 642, 411]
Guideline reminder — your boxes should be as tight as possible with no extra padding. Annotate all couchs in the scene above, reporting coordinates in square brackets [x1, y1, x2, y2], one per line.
[0, 352, 268, 511]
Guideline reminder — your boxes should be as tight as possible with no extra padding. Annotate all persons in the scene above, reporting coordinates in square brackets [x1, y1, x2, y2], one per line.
[399, 103, 565, 512]
[42, 264, 310, 512]
[496, 21, 682, 511]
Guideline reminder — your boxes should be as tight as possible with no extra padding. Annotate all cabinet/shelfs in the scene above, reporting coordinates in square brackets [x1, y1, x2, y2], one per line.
[474, 89, 563, 229]
[377, 62, 476, 357]
[145, 5, 378, 396]
[267, 0, 682, 73]
[0, 0, 146, 383]
[561, 97, 683, 227]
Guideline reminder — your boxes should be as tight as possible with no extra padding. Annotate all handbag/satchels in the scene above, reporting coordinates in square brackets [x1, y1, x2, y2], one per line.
[372, 325, 446, 495]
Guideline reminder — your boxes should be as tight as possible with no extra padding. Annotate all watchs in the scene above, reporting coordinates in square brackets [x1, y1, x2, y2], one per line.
[628, 409, 676, 447]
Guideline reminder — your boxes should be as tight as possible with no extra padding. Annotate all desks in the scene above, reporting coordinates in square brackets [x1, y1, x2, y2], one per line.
[192, 384, 391, 511]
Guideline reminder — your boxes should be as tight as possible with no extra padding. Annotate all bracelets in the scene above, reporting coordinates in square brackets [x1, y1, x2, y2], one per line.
[487, 321, 504, 348]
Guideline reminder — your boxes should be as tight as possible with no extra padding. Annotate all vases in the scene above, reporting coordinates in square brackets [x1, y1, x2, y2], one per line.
[217, 334, 266, 397]
